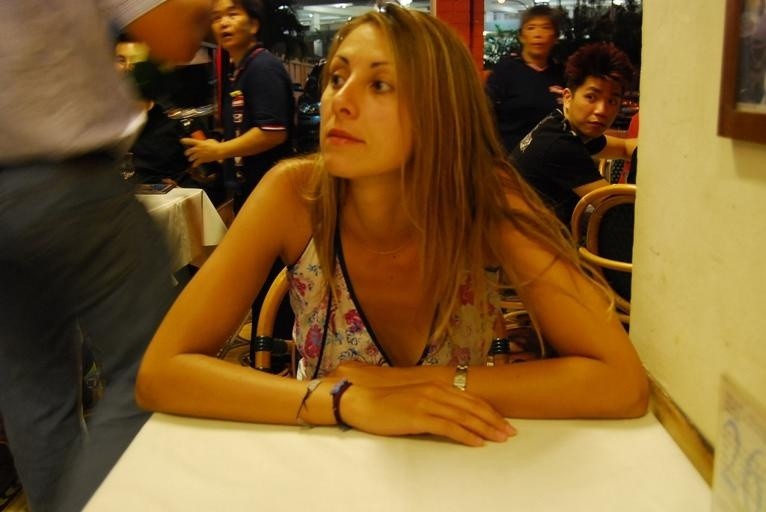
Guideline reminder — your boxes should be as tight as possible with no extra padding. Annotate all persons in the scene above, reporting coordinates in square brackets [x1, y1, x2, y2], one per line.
[133, 0, 650, 446]
[114, 31, 227, 208]
[0, 1, 180, 512]
[179, 0, 294, 359]
[485, 4, 560, 153]
[507, 42, 636, 231]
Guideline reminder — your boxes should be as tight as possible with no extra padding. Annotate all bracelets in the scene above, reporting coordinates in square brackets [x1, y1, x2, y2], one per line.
[330, 378, 351, 429]
[296, 379, 323, 427]
[453, 362, 469, 391]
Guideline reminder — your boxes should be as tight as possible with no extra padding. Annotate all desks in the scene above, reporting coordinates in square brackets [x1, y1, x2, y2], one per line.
[133, 184, 210, 283]
[84, 364, 715, 512]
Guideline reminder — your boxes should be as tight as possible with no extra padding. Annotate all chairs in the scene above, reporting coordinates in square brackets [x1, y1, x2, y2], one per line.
[573, 176, 637, 328]
[252, 264, 510, 375]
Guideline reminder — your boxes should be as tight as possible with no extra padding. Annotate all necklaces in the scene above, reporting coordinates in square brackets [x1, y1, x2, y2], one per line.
[345, 228, 418, 255]
